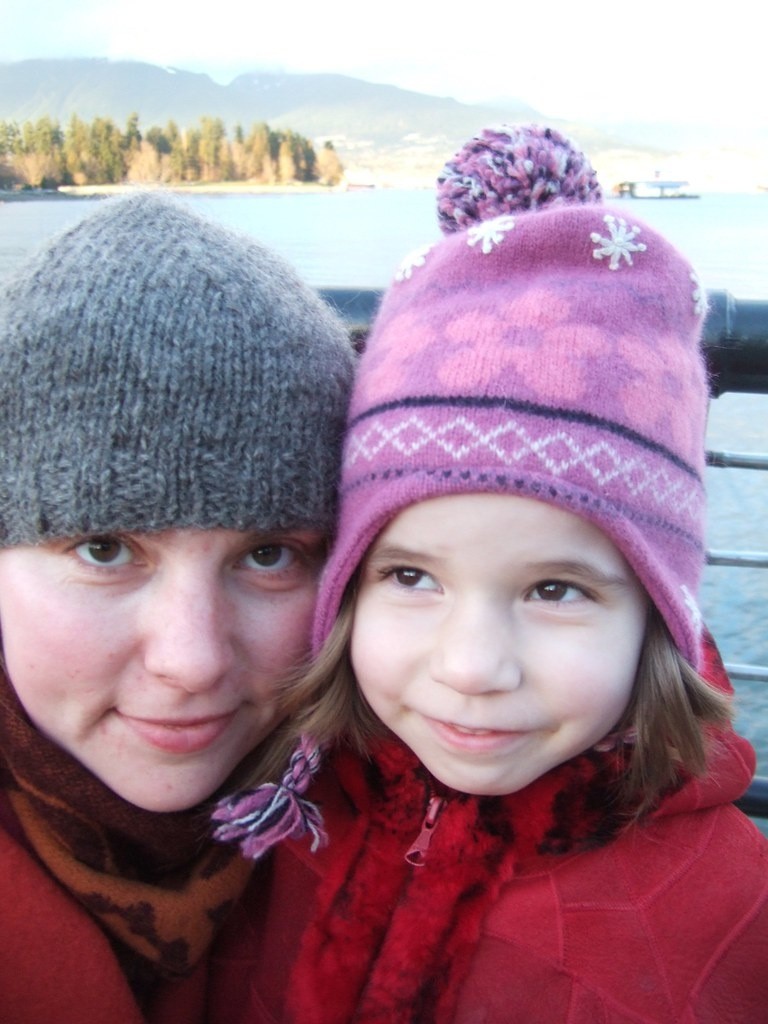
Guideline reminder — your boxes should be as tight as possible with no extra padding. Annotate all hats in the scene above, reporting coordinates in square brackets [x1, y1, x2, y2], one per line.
[0, 195, 357, 542]
[212, 129, 707, 859]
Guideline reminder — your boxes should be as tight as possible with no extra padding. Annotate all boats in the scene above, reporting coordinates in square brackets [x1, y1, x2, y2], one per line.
[348, 183, 375, 192]
[616, 179, 702, 202]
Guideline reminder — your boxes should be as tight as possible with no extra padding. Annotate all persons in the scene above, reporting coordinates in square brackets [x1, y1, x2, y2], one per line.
[1, 190, 360, 1024]
[206, 125, 767, 1024]
